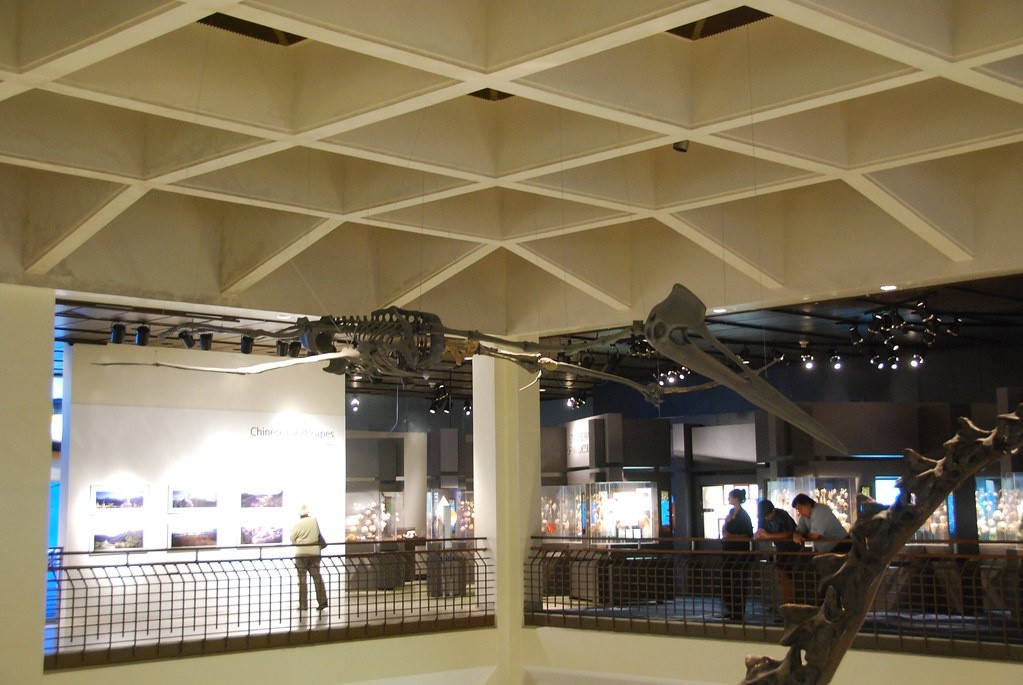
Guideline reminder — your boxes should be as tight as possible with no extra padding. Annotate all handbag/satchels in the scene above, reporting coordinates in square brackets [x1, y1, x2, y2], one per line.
[318, 534, 327, 548]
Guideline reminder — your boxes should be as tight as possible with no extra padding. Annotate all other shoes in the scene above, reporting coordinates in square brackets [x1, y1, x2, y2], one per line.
[298, 606, 307, 610]
[316, 602, 328, 610]
[721, 611, 742, 619]
[775, 618, 792, 623]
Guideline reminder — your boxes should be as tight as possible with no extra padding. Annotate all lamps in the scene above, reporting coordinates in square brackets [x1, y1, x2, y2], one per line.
[178, 330, 195, 349]
[849, 327, 864, 345]
[674, 140, 689, 153]
[867, 301, 963, 369]
[288, 341, 301, 357]
[827, 353, 841, 370]
[276, 340, 288, 356]
[352, 394, 360, 411]
[199, 332, 212, 350]
[653, 365, 691, 386]
[241, 335, 254, 354]
[567, 394, 586, 410]
[738, 346, 751, 365]
[135, 326, 149, 346]
[557, 339, 656, 374]
[429, 404, 438, 413]
[443, 401, 453, 414]
[800, 352, 814, 369]
[110, 324, 126, 344]
[462, 401, 472, 416]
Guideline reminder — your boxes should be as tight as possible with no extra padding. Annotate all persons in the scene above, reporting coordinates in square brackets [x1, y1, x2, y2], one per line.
[754, 499, 805, 622]
[290, 503, 328, 611]
[791, 493, 854, 554]
[722, 488, 753, 620]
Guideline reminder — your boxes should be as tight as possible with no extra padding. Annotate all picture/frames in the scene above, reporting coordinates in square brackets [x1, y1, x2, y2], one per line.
[236, 524, 286, 547]
[89, 529, 148, 554]
[167, 484, 220, 513]
[238, 489, 285, 513]
[90, 485, 147, 513]
[167, 524, 222, 549]
